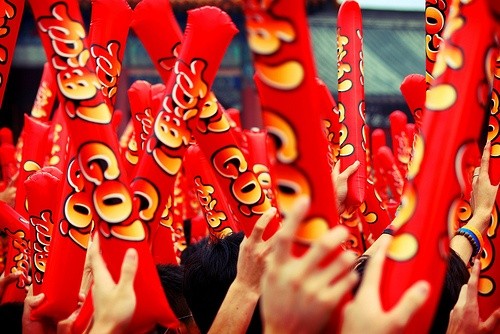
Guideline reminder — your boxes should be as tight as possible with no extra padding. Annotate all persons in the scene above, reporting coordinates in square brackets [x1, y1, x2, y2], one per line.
[0, 138, 500, 334]
